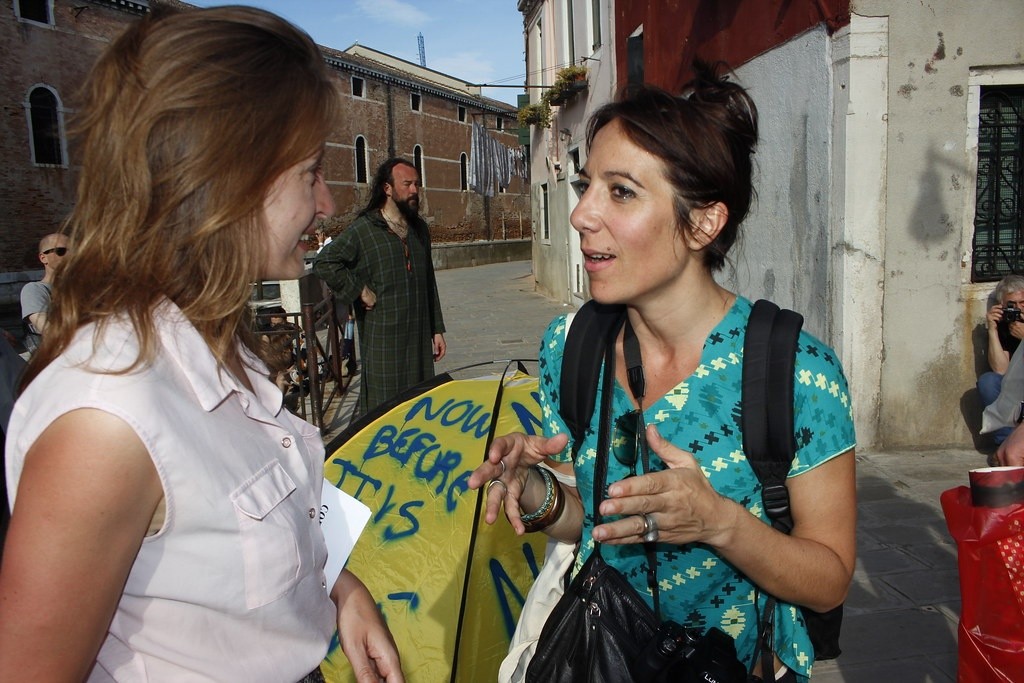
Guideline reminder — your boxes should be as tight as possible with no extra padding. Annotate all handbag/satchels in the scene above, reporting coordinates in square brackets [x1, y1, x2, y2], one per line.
[525, 551, 763, 683]
[496, 538, 578, 682]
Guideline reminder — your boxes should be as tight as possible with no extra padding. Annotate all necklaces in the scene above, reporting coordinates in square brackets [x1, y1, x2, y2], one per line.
[382, 208, 411, 272]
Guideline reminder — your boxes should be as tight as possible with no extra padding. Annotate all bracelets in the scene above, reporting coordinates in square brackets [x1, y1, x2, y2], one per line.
[503, 464, 566, 533]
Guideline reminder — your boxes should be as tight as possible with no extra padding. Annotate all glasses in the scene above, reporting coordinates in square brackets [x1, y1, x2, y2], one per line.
[44, 247, 67, 257]
[612, 412, 640, 519]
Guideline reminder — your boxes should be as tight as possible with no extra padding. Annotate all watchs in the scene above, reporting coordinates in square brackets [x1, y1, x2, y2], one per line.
[1013, 400, 1024, 427]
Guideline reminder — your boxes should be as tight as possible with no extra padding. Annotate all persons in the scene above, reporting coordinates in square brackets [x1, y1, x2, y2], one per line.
[978, 274, 1024, 467]
[314, 156, 446, 417]
[467, 57, 856, 683]
[0, 5, 406, 683]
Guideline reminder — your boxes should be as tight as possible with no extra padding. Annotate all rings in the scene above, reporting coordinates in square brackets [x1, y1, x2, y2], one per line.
[486, 480, 507, 496]
[639, 512, 659, 541]
[499, 461, 504, 474]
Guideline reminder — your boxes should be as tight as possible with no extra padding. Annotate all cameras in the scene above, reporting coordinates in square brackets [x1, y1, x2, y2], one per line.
[998, 301, 1021, 322]
